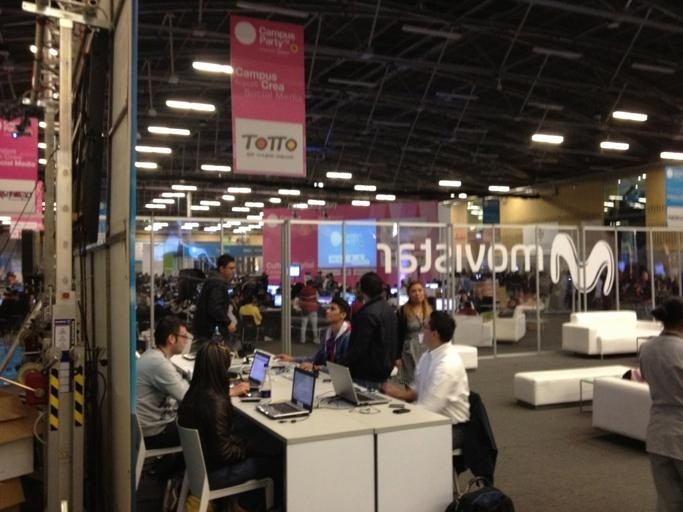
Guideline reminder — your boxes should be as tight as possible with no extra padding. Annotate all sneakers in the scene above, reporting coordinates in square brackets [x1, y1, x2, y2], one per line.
[264, 334, 274, 342]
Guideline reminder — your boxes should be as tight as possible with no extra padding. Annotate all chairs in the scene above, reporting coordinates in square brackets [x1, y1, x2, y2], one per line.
[241, 314, 260, 346]
[132, 412, 183, 493]
[174, 416, 275, 512]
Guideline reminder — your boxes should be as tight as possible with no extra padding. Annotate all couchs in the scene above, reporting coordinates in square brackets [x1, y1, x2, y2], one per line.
[453, 315, 493, 347]
[493, 311, 527, 342]
[579, 376, 654, 445]
[561, 310, 663, 360]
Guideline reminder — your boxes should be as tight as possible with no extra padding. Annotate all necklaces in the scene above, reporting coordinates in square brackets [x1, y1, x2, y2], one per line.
[157, 345, 168, 359]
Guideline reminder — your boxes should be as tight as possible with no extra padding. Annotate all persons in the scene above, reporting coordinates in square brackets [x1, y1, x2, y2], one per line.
[3, 271, 23, 302]
[395, 280, 434, 403]
[232, 273, 272, 303]
[273, 296, 352, 371]
[238, 294, 274, 342]
[136, 270, 198, 315]
[378, 310, 471, 450]
[135, 315, 206, 460]
[565, 263, 683, 311]
[638, 296, 683, 512]
[298, 280, 321, 345]
[275, 280, 303, 318]
[192, 254, 242, 352]
[177, 342, 282, 512]
[304, 271, 344, 296]
[434, 269, 555, 321]
[349, 272, 407, 385]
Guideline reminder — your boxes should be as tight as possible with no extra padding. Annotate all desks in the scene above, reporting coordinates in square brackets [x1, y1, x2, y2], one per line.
[170, 349, 454, 512]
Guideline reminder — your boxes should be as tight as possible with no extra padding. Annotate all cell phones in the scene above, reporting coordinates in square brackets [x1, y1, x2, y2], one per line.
[393, 408, 409, 413]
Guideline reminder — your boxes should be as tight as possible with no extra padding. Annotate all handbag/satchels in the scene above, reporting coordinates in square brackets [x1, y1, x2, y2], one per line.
[442, 473, 517, 508]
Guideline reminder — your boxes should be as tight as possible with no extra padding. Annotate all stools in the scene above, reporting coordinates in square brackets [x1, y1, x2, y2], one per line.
[450, 343, 478, 370]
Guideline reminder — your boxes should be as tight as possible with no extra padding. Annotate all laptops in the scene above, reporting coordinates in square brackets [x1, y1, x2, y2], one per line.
[256, 366, 316, 420]
[327, 360, 392, 405]
[228, 351, 270, 391]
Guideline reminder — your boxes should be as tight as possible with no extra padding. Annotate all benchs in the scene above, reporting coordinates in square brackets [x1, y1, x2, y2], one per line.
[513, 364, 635, 407]
